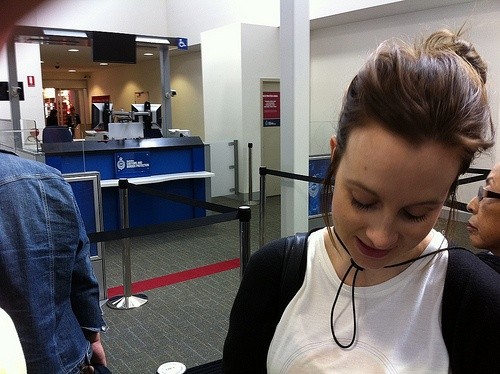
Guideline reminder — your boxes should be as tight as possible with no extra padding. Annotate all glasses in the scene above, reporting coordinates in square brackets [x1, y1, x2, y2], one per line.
[478, 185, 500, 201]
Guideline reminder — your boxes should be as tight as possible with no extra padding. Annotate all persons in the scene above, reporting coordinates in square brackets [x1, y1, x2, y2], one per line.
[178, 28, 500, 374]
[0, 0, 110, 374]
[465, 164, 500, 272]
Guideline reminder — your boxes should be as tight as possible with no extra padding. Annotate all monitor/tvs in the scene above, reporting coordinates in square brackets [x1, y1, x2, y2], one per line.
[0, 81, 24, 101]
[108, 122, 144, 140]
[91, 103, 114, 130]
[93, 32, 137, 64]
[131, 104, 162, 127]
